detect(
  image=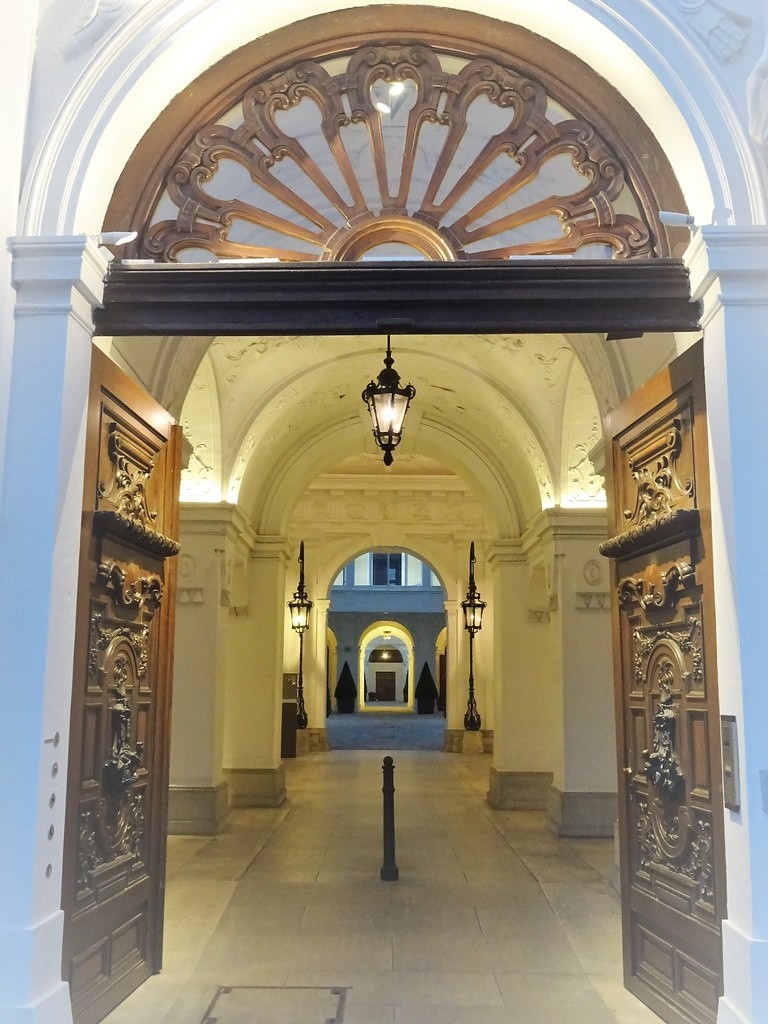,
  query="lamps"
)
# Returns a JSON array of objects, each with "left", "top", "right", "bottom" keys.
[
  {"left": 459, "top": 541, "right": 487, "bottom": 639},
  {"left": 288, "top": 540, "right": 314, "bottom": 636},
  {"left": 362, "top": 334, "right": 417, "bottom": 467}
]
